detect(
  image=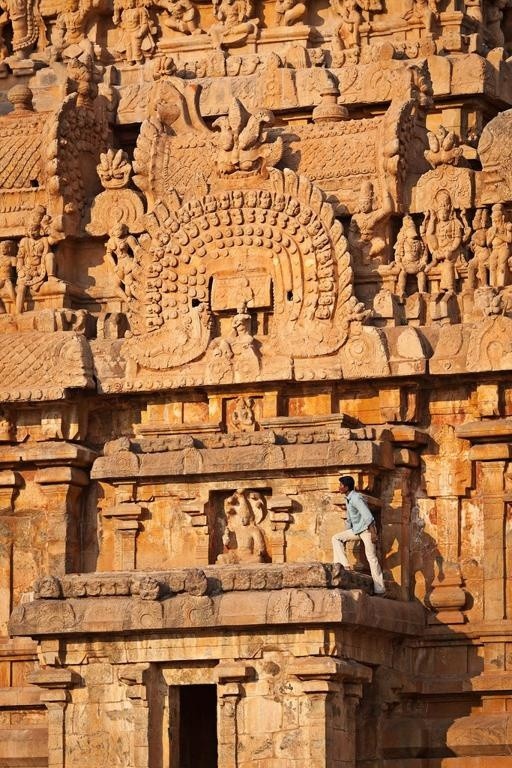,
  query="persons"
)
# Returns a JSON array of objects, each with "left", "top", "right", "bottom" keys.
[{"left": 331, "top": 476, "right": 386, "bottom": 597}]
